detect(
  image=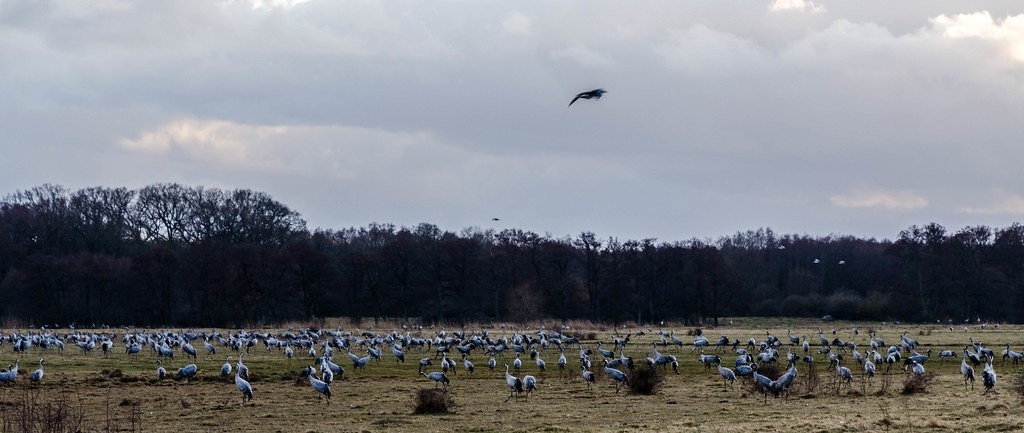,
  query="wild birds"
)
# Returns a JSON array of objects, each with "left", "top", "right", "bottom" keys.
[
  {"left": 491, "top": 217, "right": 500, "bottom": 222},
  {"left": 569, "top": 89, "right": 608, "bottom": 106},
  {"left": 0, "top": 319, "right": 1024, "bottom": 413}
]
